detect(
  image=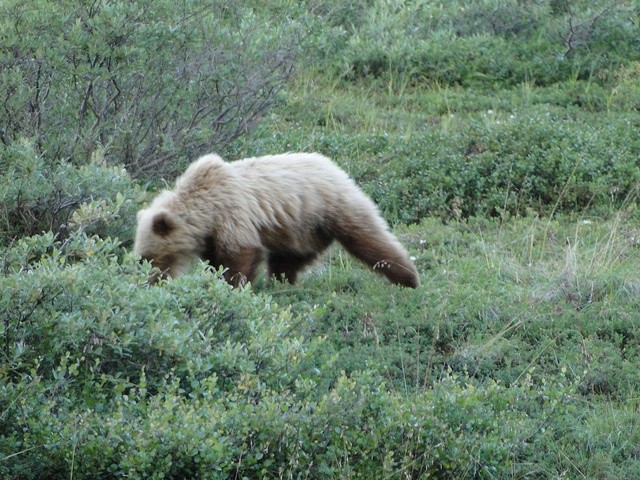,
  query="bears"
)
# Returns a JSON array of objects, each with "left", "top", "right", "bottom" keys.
[{"left": 133, "top": 150, "right": 423, "bottom": 289}]
